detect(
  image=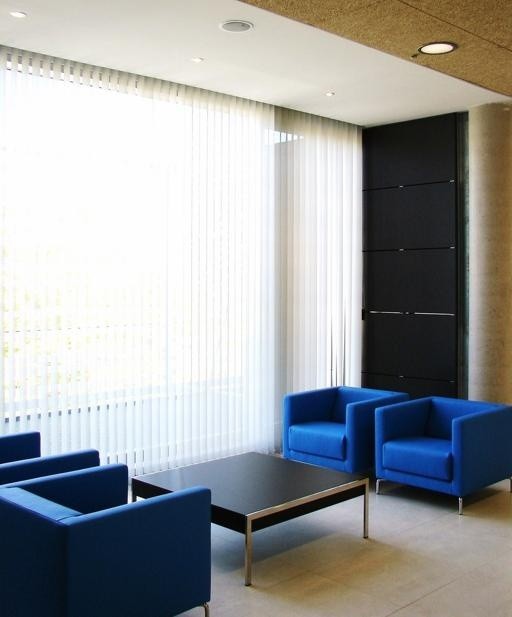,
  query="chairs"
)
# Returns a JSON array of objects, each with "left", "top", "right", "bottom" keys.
[
  {"left": 0, "top": 430, "right": 212, "bottom": 616},
  {"left": 281, "top": 386, "right": 511, "bottom": 514}
]
